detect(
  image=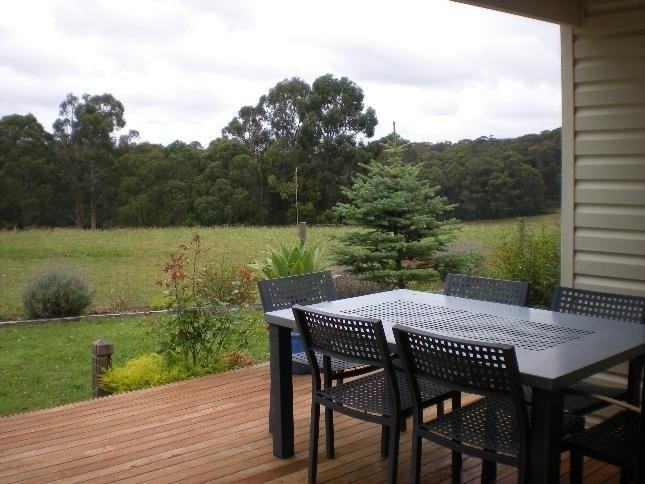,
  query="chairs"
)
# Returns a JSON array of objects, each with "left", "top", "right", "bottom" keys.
[{"left": 256, "top": 269, "right": 645, "bottom": 483}]
